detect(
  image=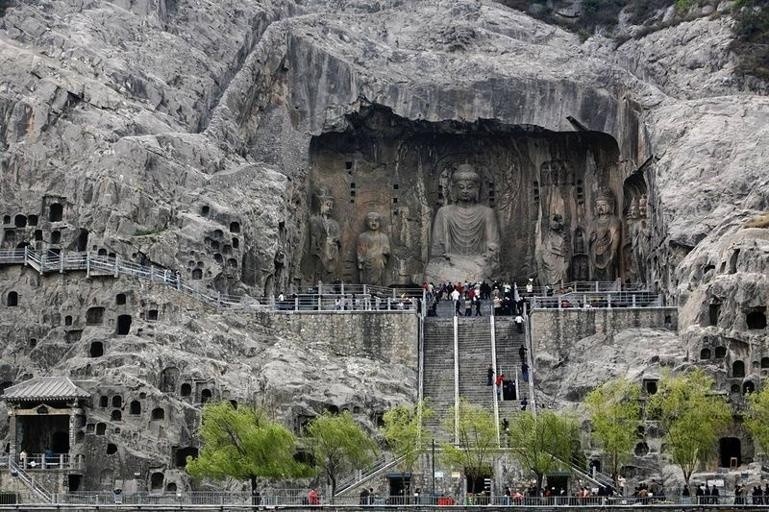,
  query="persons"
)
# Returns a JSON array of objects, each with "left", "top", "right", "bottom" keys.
[
  {"left": 631, "top": 480, "right": 667, "bottom": 506},
  {"left": 629, "top": 198, "right": 645, "bottom": 289},
  {"left": 634, "top": 195, "right": 647, "bottom": 290},
  {"left": 587, "top": 187, "right": 621, "bottom": 290},
  {"left": 277, "top": 292, "right": 299, "bottom": 310},
  {"left": 308, "top": 185, "right": 346, "bottom": 284},
  {"left": 399, "top": 487, "right": 421, "bottom": 509},
  {"left": 354, "top": 211, "right": 390, "bottom": 289},
  {"left": 308, "top": 488, "right": 319, "bottom": 512},
  {"left": 334, "top": 295, "right": 418, "bottom": 310},
  {"left": 683, "top": 484, "right": 720, "bottom": 504},
  {"left": 485, "top": 344, "right": 532, "bottom": 433},
  {"left": 559, "top": 485, "right": 614, "bottom": 505},
  {"left": 504, "top": 484, "right": 556, "bottom": 505},
  {"left": 359, "top": 487, "right": 375, "bottom": 509},
  {"left": 2, "top": 440, "right": 38, "bottom": 470},
  {"left": 422, "top": 281, "right": 607, "bottom": 334},
  {"left": 733, "top": 484, "right": 768, "bottom": 505},
  {"left": 421, "top": 162, "right": 503, "bottom": 287},
  {"left": 540, "top": 211, "right": 572, "bottom": 287}
]
